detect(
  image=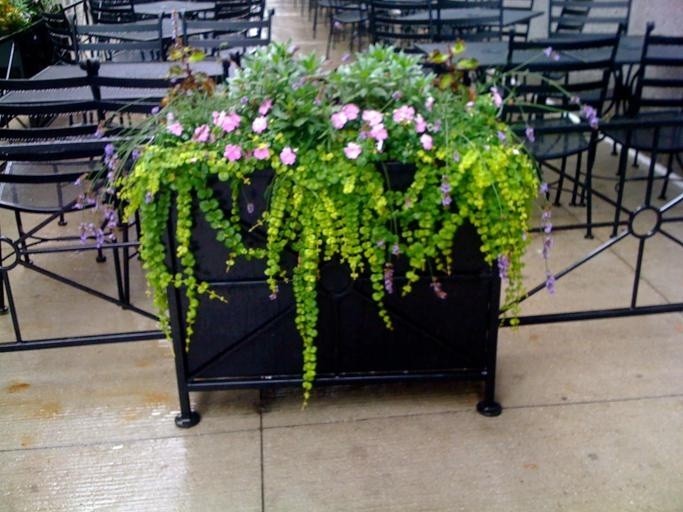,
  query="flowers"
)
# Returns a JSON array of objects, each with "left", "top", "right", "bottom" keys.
[{"left": 71, "top": 5, "right": 597, "bottom": 414}]
